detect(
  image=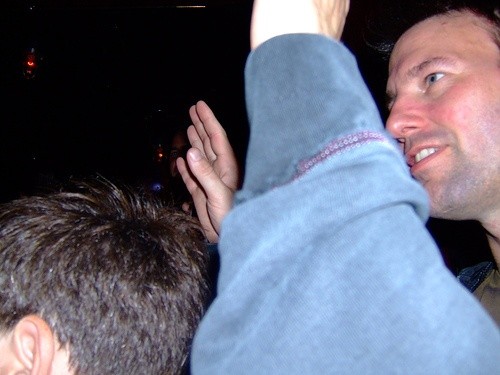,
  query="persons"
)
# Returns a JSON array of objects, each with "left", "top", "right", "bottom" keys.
[
  {"left": 175, "top": 1, "right": 500, "bottom": 328},
  {"left": 181, "top": 199, "right": 200, "bottom": 223},
  {"left": 1, "top": 170, "right": 208, "bottom": 375},
  {"left": 192, "top": 1, "right": 500, "bottom": 375}
]
